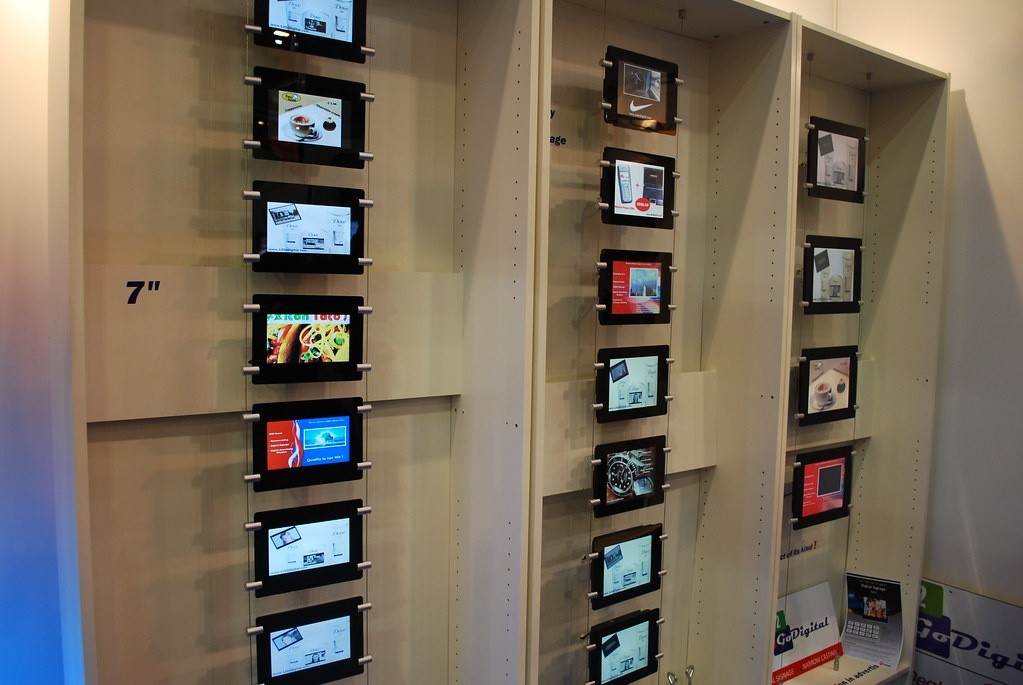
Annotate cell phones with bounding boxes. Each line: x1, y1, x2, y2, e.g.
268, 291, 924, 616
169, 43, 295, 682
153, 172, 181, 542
617, 165, 633, 203
